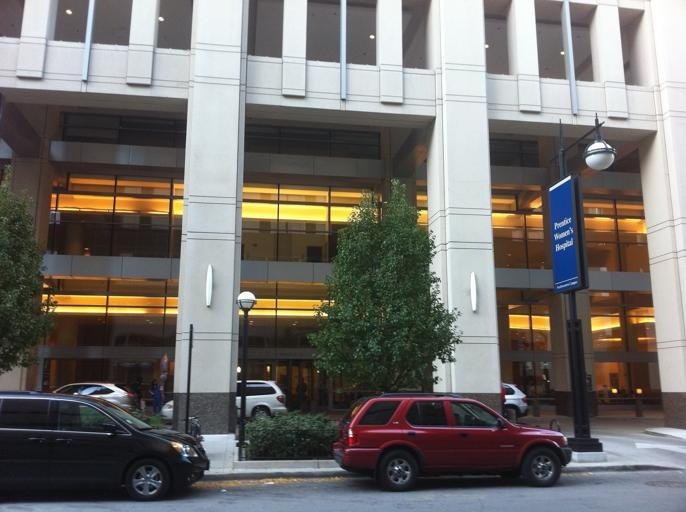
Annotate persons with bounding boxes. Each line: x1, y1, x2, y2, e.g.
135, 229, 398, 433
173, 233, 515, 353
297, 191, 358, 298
146, 376, 162, 411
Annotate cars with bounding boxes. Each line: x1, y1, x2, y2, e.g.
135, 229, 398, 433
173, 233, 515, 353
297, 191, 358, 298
0, 381, 210, 502
331, 383, 573, 492
235, 377, 289, 423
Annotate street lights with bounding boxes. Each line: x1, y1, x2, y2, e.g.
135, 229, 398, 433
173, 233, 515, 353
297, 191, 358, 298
235, 290, 257, 462
548, 112, 618, 453
